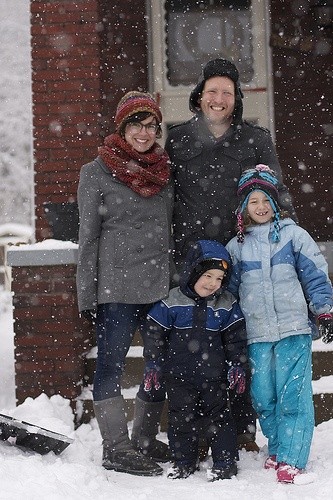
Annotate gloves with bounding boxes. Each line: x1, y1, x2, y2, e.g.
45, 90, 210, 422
227, 366, 246, 394
317, 314, 333, 344
143, 362, 161, 391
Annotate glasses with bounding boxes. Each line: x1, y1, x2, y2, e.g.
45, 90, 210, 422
127, 121, 159, 132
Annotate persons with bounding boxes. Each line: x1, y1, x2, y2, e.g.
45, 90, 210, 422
144, 240, 252, 481
77, 91, 181, 476
224, 164, 333, 483
163, 59, 298, 463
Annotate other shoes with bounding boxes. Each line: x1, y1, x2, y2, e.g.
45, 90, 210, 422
198, 447, 208, 462
237, 441, 260, 454
167, 462, 200, 479
208, 463, 238, 482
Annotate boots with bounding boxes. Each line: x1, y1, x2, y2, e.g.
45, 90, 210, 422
93, 395, 164, 476
131, 395, 171, 463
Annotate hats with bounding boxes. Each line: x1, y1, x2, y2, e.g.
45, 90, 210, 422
234, 164, 281, 244
115, 91, 163, 139
189, 58, 244, 128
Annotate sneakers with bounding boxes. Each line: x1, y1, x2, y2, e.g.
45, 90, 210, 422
276, 463, 302, 482
265, 455, 279, 470
